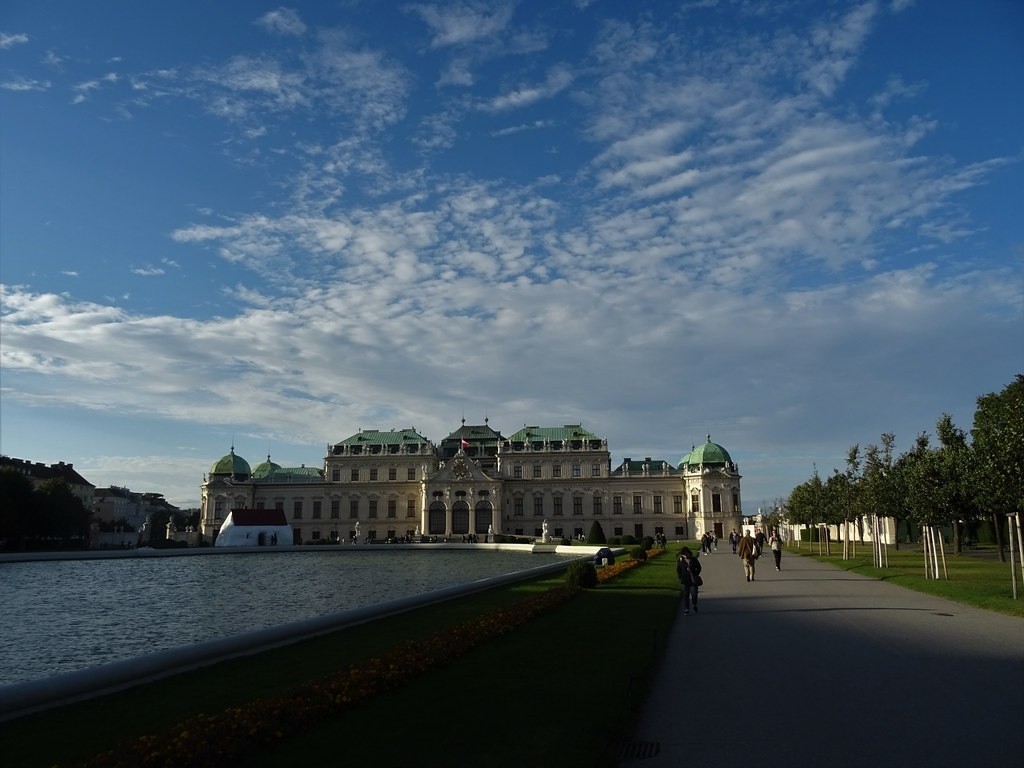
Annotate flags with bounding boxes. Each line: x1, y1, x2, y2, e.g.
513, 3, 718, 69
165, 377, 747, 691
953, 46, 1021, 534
461, 439, 468, 447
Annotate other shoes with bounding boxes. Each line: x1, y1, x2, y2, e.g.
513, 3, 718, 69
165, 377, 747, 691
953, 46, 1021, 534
732, 552, 737, 554
702, 551, 708, 556
759, 555, 762, 558
746, 576, 750, 583
683, 610, 691, 616
709, 552, 713, 556
692, 607, 699, 614
752, 578, 755, 581
775, 566, 780, 571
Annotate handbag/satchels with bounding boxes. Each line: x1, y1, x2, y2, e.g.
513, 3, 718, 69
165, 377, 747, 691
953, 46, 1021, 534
751, 537, 759, 557
689, 573, 703, 587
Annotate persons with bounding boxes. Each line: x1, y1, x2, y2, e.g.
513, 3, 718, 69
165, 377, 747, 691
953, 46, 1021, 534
270, 533, 277, 546
769, 530, 784, 571
462, 533, 479, 543
756, 529, 768, 558
655, 532, 666, 549
578, 534, 585, 542
337, 535, 345, 544
677, 547, 703, 616
562, 533, 564, 540
121, 540, 132, 550
701, 531, 718, 555
484, 534, 488, 543
729, 529, 743, 554
353, 536, 357, 545
738, 530, 760, 582
100, 541, 107, 550
366, 534, 447, 543
